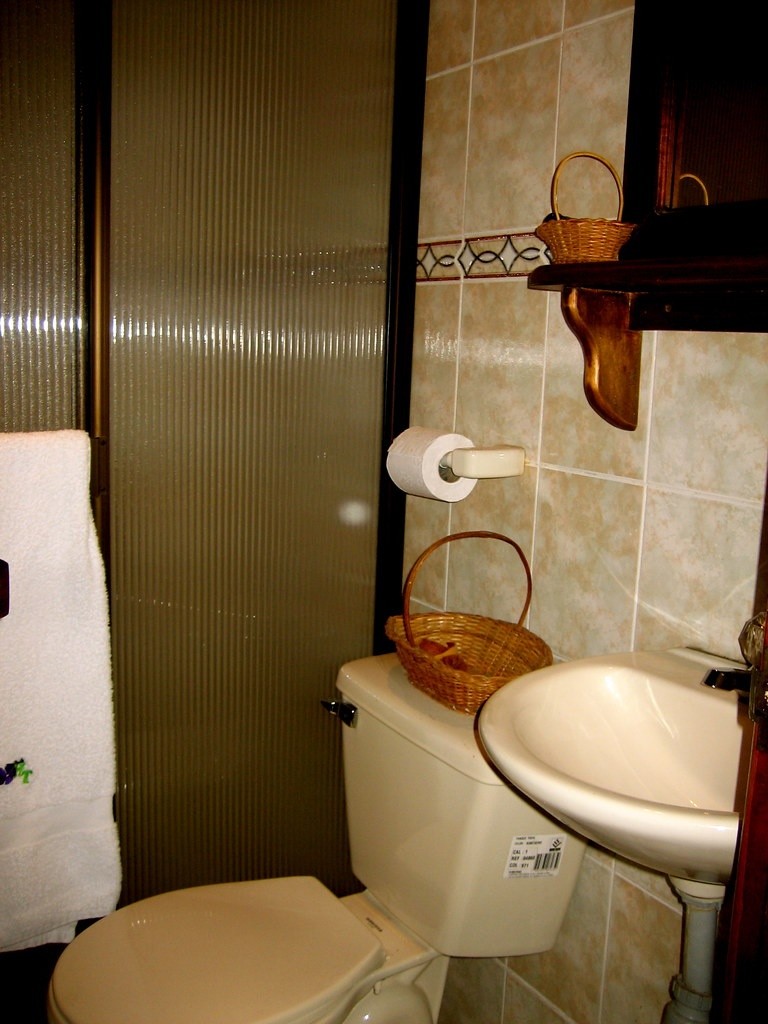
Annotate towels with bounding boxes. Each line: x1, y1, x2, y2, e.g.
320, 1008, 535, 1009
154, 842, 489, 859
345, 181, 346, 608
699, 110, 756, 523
0, 428, 124, 955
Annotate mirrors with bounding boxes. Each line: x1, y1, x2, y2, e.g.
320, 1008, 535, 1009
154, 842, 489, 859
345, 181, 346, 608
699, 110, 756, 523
619, 0, 768, 332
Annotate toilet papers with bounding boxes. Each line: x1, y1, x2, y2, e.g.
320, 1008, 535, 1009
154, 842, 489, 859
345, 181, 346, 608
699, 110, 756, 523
386, 425, 478, 504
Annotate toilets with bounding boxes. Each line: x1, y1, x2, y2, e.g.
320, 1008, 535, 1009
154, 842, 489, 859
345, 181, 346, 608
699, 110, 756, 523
46, 646, 588, 1024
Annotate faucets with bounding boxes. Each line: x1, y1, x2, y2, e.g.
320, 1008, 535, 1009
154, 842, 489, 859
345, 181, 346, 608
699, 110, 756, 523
699, 666, 755, 693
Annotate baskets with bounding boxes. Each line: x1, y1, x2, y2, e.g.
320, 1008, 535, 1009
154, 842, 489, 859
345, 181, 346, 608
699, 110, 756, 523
535, 151, 636, 263
386, 529, 553, 717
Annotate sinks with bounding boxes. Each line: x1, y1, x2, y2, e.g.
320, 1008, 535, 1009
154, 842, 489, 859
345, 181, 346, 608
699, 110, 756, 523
473, 647, 758, 887
